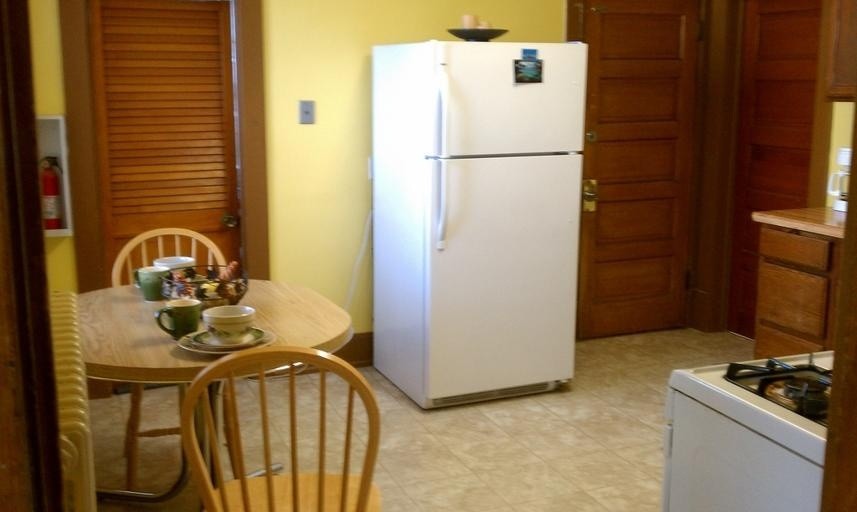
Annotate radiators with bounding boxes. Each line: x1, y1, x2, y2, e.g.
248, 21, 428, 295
47, 288, 98, 512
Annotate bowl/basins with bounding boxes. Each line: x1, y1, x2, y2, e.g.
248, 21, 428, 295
158, 265, 250, 309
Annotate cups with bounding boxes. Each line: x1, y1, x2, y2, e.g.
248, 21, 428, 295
132, 266, 168, 300
153, 256, 196, 268
201, 305, 256, 343
155, 298, 202, 340
460, 15, 477, 30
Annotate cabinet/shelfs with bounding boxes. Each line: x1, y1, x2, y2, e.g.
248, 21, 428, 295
748, 206, 845, 358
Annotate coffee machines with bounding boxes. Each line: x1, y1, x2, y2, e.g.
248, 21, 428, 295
824, 148, 852, 214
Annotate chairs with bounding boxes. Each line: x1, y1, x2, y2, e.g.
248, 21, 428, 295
182, 350, 382, 512
111, 226, 264, 489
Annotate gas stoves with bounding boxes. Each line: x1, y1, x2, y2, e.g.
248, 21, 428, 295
661, 349, 835, 467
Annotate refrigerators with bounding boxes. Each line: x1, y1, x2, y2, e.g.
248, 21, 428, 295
370, 40, 589, 409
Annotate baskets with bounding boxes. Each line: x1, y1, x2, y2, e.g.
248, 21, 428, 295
158, 264, 248, 319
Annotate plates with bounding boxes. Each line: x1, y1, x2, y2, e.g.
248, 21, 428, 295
446, 28, 507, 43
177, 329, 276, 355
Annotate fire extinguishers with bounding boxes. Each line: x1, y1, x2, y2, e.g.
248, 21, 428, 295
39, 157, 63, 229
